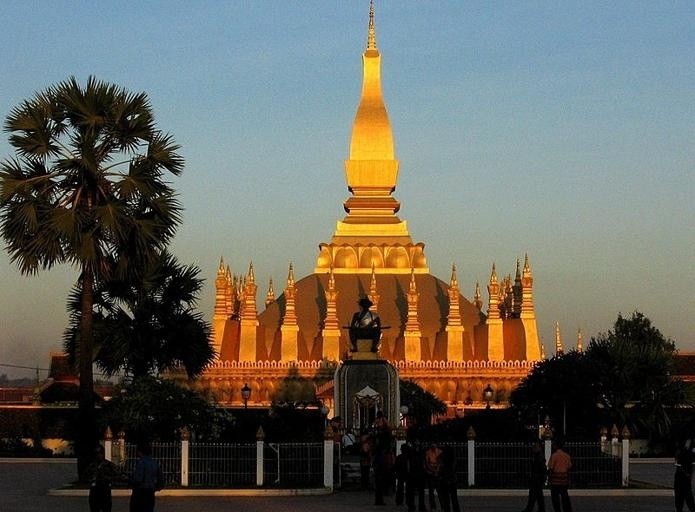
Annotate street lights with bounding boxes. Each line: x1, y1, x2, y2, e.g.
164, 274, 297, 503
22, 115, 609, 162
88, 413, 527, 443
241, 382, 251, 407
483, 382, 494, 409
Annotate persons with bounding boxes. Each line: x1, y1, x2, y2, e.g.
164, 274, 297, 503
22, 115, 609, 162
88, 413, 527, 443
369, 444, 389, 506
341, 429, 357, 456
124, 441, 164, 512
383, 445, 396, 498
405, 442, 428, 511
436, 445, 462, 512
395, 443, 409, 506
425, 439, 444, 512
347, 297, 381, 353
547, 439, 573, 512
672, 439, 695, 512
85, 444, 125, 512
372, 411, 388, 427
359, 435, 375, 492
522, 440, 548, 511
